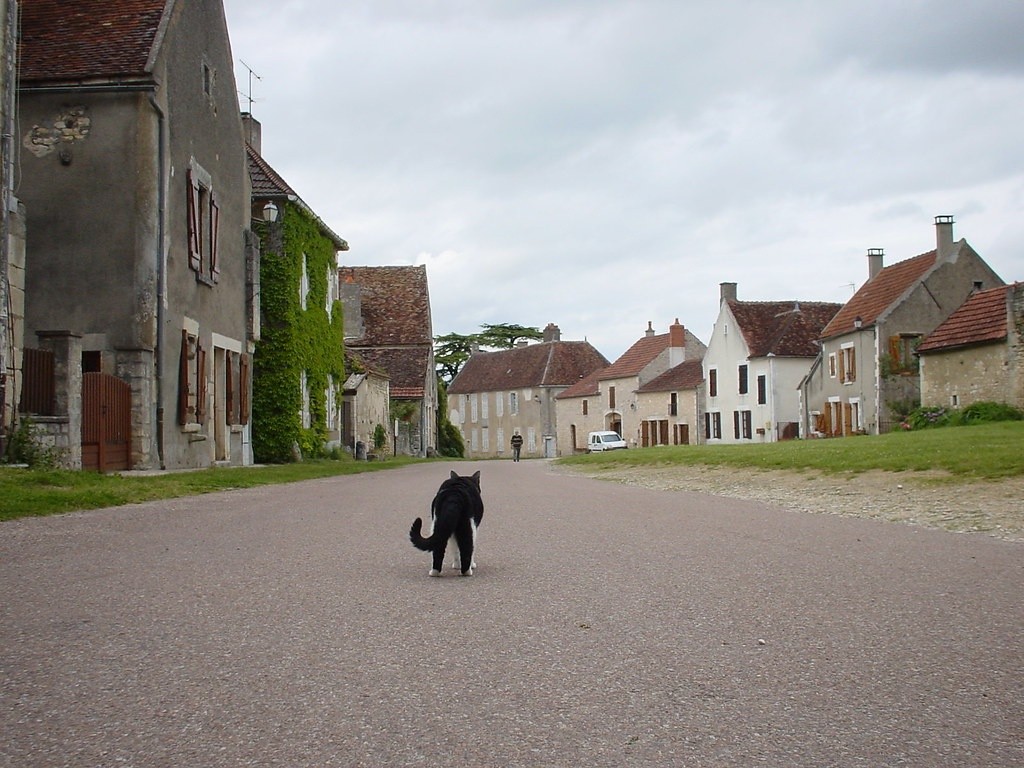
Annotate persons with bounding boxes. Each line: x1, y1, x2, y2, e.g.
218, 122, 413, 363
511, 431, 523, 463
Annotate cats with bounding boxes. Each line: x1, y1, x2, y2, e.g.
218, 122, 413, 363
408, 470, 484, 577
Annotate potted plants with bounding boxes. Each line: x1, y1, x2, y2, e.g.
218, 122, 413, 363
367, 449, 380, 463
372, 424, 388, 461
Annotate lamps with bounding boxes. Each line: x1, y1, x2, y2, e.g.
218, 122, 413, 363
534, 395, 541, 405
251, 200, 278, 226
853, 316, 876, 341
631, 404, 636, 411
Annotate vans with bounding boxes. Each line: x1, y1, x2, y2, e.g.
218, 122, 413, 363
588, 431, 628, 453
